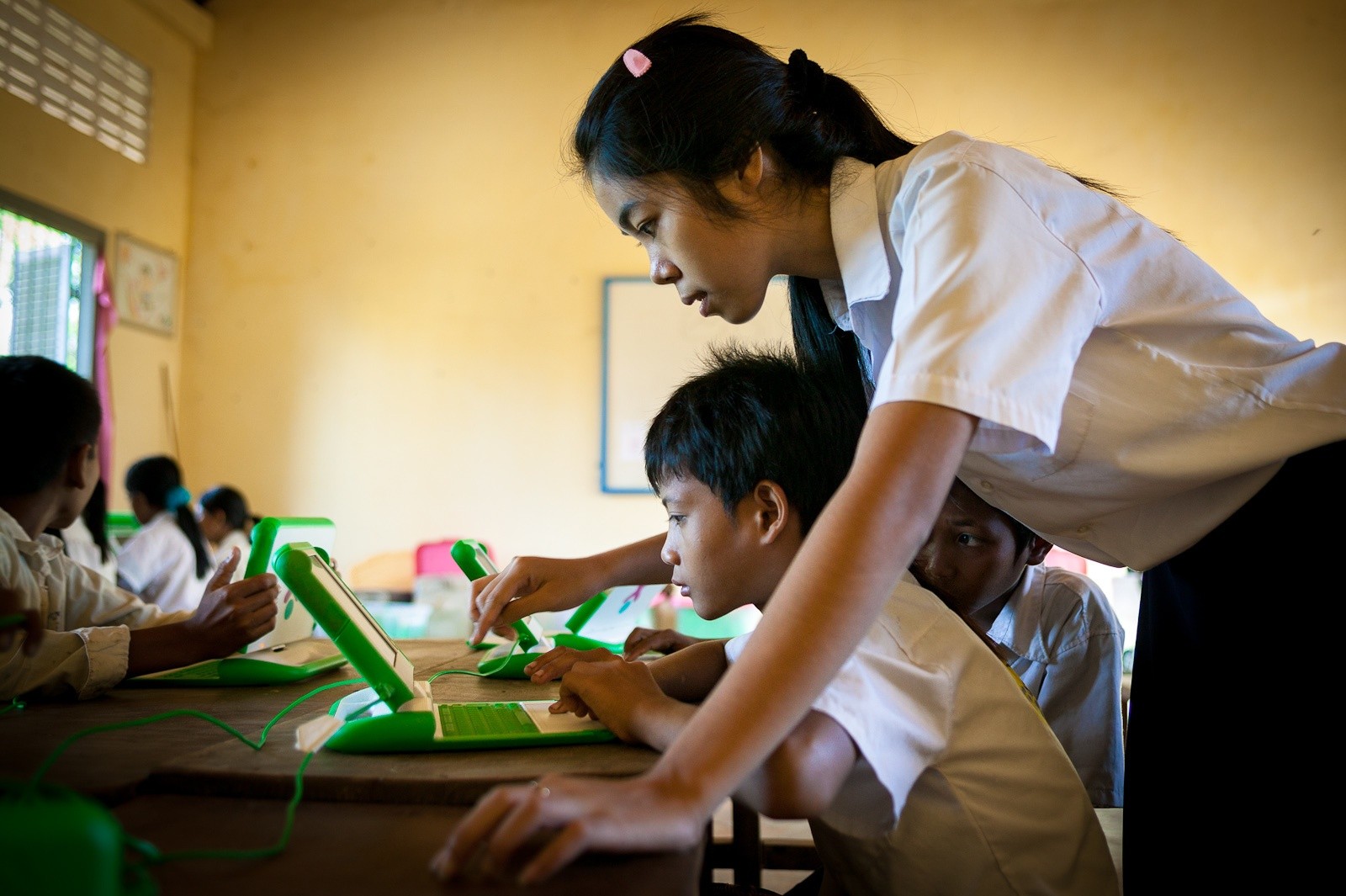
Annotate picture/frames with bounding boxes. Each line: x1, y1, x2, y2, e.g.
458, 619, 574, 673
110, 228, 181, 336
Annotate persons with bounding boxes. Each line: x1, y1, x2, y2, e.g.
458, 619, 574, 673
42, 475, 118, 585
427, 10, 1346, 896
549, 356, 1124, 896
0, 354, 280, 699
197, 484, 264, 585
118, 454, 216, 613
524, 477, 1122, 810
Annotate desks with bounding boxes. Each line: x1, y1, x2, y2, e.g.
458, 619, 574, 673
0, 637, 706, 896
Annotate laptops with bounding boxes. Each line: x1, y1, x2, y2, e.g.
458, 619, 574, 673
103, 512, 668, 755
